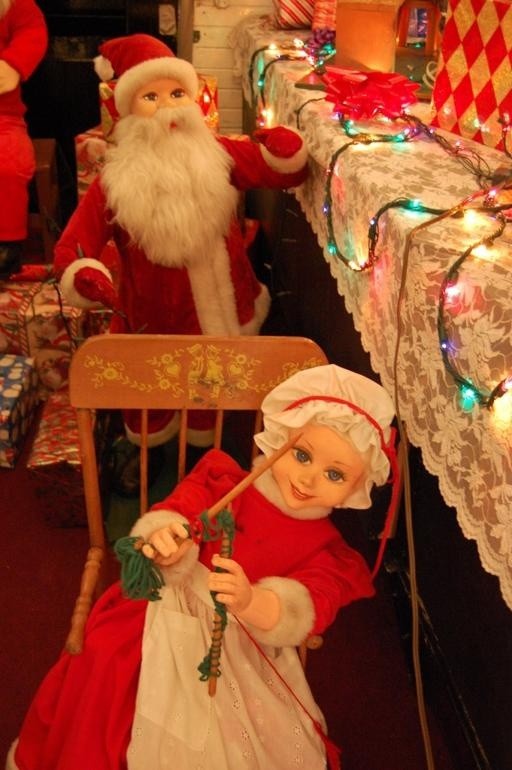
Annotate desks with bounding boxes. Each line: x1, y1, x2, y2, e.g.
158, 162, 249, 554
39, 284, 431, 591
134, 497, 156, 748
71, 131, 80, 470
241, 12, 512, 768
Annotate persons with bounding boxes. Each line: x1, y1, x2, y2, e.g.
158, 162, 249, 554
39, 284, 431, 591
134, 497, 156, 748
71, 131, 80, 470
1, 0, 50, 252
2, 362, 398, 770
48, 31, 311, 503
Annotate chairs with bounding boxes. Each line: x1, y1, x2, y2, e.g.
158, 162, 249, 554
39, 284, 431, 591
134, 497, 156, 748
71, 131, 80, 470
66, 335, 332, 675
